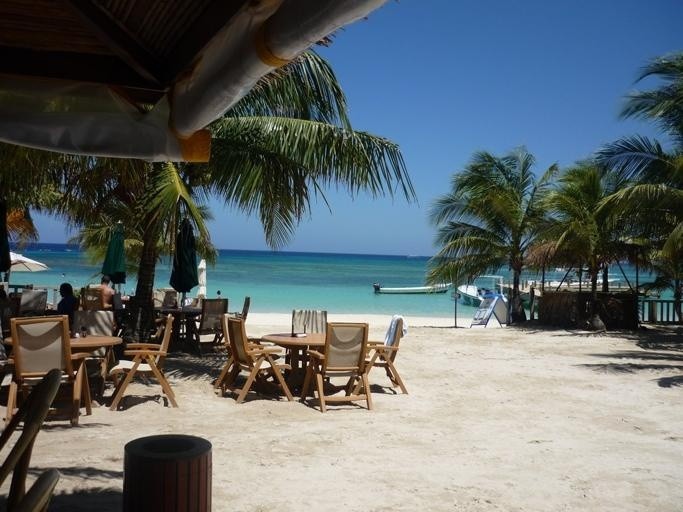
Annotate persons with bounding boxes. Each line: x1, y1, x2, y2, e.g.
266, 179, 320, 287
96, 274, 115, 309
44, 281, 75, 328
215, 289, 223, 299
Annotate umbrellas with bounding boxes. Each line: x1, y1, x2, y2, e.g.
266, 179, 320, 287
196, 256, 208, 303
100, 220, 126, 292
166, 217, 198, 307
7, 250, 50, 285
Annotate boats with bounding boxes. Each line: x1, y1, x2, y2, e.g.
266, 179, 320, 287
457, 284, 495, 305
373, 282, 453, 294
527, 278, 620, 289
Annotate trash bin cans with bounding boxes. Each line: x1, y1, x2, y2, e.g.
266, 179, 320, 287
122, 434, 213, 512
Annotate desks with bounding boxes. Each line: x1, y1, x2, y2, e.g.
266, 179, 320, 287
4, 335, 122, 407
261, 333, 329, 388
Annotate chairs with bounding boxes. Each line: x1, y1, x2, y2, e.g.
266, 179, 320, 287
0, 368, 63, 511
345, 314, 407, 395
214, 312, 295, 404
0, 284, 251, 359
109, 314, 179, 412
286, 308, 330, 386
5, 314, 91, 428
300, 322, 373, 414
